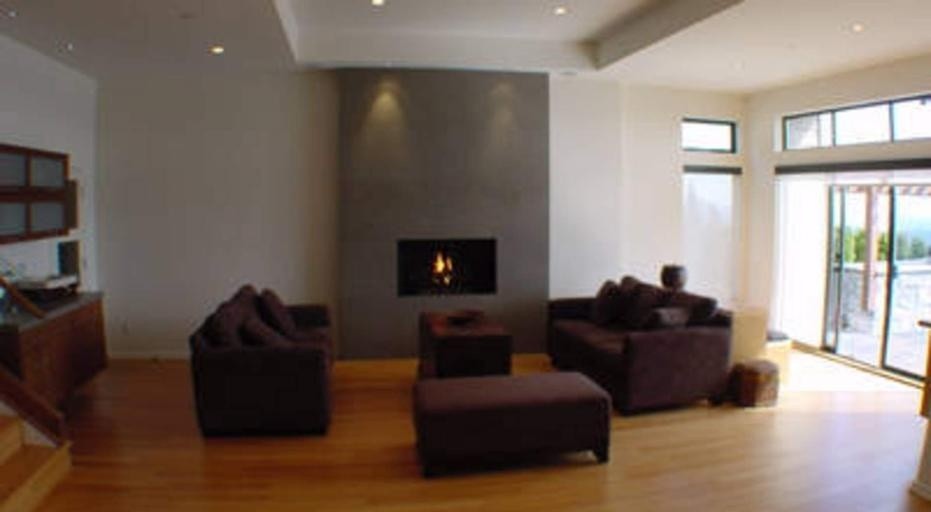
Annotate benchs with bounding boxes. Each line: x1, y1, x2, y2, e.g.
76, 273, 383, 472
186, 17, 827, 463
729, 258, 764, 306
412, 370, 611, 478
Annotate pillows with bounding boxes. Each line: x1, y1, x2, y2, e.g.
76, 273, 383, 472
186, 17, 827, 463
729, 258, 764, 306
593, 280, 628, 325
257, 288, 296, 340
640, 307, 693, 329
239, 316, 289, 344
621, 284, 670, 329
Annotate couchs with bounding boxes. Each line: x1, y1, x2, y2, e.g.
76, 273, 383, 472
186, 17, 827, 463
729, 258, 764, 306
546, 274, 734, 416
189, 283, 330, 438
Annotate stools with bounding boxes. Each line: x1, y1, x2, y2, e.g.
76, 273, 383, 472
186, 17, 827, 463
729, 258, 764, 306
736, 358, 781, 409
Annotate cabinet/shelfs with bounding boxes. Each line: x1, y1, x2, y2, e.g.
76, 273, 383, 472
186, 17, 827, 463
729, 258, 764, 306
0, 291, 107, 407
